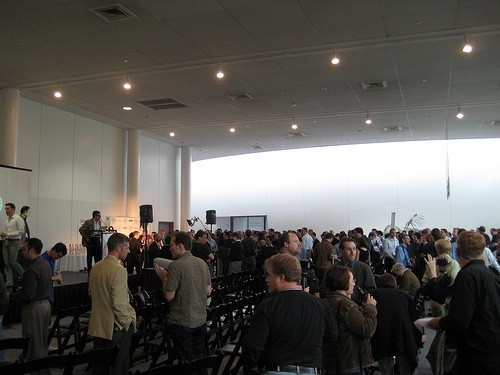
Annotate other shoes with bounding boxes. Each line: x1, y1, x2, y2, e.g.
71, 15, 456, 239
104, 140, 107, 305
47, 346, 58, 352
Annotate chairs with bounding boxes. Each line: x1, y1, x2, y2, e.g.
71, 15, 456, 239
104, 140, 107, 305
0, 262, 446, 375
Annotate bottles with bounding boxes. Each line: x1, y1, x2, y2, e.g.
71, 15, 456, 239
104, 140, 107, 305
57, 269, 62, 284
356, 285, 368, 306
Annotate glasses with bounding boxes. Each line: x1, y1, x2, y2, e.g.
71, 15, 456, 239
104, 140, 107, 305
351, 279, 356, 283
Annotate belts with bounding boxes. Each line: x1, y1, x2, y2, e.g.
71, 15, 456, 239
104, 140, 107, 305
265, 364, 323, 375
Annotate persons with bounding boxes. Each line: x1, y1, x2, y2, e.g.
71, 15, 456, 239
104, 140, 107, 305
156, 231, 211, 375
320, 237, 377, 307
0, 272, 8, 361
88, 233, 137, 375
0, 203, 24, 290
320, 265, 377, 375
127, 230, 180, 275
428, 231, 500, 375
206, 228, 283, 276
280, 231, 301, 255
295, 226, 370, 296
79, 211, 103, 276
245, 254, 337, 375
370, 273, 418, 375
477, 226, 500, 275
19, 206, 30, 264
15, 238, 51, 375
369, 228, 465, 347
393, 264, 419, 300
40, 242, 67, 349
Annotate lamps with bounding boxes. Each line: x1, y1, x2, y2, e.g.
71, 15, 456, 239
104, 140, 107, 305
462, 35, 473, 53
366, 113, 372, 125
455, 105, 463, 118
186, 216, 206, 230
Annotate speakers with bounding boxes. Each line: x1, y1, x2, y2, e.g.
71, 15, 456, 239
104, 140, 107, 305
139, 204, 153, 223
206, 210, 216, 224
